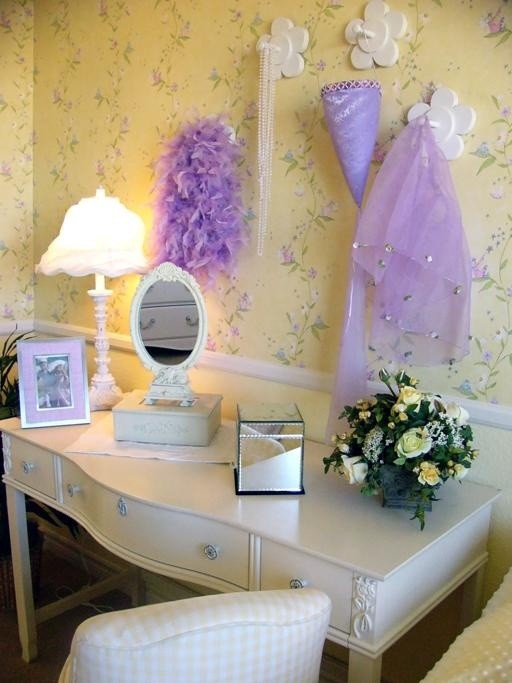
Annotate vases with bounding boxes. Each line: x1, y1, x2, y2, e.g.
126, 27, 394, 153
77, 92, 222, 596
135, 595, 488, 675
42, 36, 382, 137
379, 465, 434, 513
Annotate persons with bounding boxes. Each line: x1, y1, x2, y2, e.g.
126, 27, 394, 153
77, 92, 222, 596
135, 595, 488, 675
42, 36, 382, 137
35, 357, 70, 408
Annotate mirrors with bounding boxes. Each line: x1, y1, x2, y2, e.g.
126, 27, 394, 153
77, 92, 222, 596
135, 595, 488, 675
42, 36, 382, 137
129, 263, 208, 377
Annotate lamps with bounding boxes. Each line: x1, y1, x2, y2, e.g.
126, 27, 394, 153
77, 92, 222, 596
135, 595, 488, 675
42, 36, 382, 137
34, 185, 156, 412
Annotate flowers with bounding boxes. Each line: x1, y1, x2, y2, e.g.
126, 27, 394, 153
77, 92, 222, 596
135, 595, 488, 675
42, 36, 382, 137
322, 368, 478, 531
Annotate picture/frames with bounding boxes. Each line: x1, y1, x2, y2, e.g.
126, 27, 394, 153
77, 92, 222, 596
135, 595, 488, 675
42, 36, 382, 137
17, 336, 92, 429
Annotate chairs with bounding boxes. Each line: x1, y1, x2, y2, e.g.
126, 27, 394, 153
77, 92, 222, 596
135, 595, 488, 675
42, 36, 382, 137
57, 587, 333, 683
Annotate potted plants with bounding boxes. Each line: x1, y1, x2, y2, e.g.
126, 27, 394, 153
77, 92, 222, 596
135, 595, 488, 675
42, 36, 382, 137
1, 322, 85, 616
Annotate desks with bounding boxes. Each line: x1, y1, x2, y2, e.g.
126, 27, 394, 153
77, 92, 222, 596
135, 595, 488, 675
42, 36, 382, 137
0, 405, 506, 682
